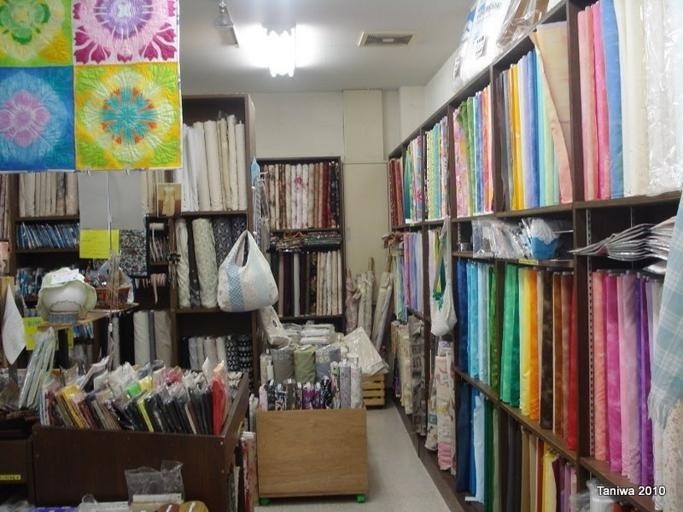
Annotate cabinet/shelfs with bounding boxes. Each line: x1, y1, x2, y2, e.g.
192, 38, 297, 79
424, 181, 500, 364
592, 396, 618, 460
23, 371, 254, 511
1, 92, 261, 395
386, 0, 683, 512
255, 157, 347, 335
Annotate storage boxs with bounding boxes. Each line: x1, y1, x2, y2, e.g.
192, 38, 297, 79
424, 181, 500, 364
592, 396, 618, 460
255, 406, 373, 503
